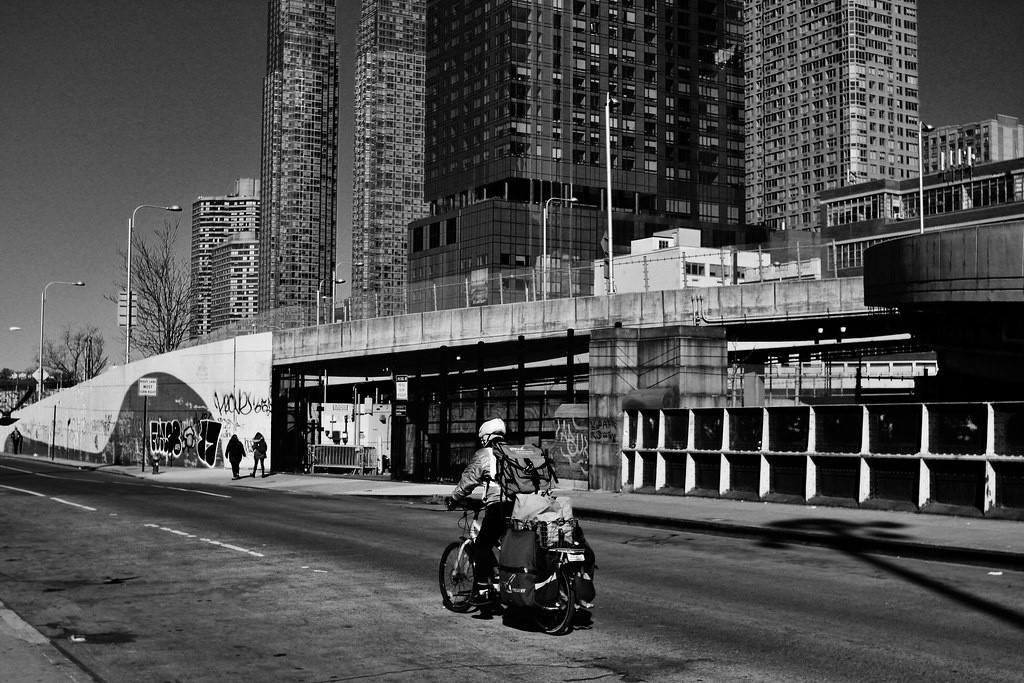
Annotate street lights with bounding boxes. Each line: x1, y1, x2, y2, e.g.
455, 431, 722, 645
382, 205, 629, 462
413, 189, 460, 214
37, 281, 85, 402
543, 198, 578, 300
603, 92, 620, 293
917, 122, 935, 233
332, 262, 365, 324
126, 205, 184, 365
317, 278, 346, 326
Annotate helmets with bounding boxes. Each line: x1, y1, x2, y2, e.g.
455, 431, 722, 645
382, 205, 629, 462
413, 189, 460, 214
477, 417, 507, 448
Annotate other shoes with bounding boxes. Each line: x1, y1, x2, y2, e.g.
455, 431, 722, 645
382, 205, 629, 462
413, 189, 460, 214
250, 473, 255, 478
231, 475, 241, 480
262, 474, 265, 478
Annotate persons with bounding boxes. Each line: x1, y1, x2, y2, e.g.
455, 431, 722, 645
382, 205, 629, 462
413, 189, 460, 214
252, 432, 267, 478
11, 428, 21, 454
225, 435, 246, 480
444, 418, 518, 608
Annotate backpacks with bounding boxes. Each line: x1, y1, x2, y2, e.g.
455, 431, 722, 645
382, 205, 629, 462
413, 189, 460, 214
491, 441, 560, 498
256, 440, 268, 454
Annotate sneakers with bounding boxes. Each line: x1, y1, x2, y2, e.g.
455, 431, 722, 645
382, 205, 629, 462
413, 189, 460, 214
467, 580, 495, 606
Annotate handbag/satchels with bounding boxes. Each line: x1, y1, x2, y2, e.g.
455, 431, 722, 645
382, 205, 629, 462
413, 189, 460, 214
511, 489, 576, 548
570, 520, 600, 610
498, 527, 569, 611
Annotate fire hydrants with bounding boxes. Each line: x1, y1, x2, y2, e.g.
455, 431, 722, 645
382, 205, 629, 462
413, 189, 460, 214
151, 456, 160, 473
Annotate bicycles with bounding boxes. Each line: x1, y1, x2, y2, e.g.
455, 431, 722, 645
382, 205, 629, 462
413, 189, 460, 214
438, 497, 586, 634
